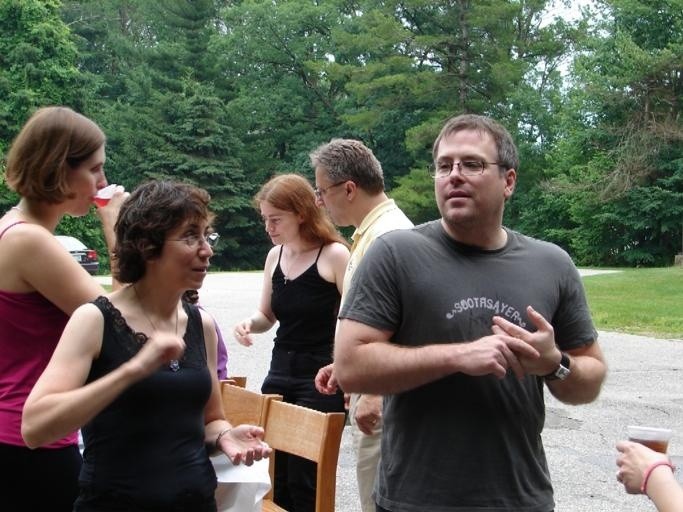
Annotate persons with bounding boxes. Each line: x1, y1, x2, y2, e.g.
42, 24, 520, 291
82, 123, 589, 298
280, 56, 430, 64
21, 179, 272, 512
332, 112, 608, 512
232, 174, 350, 512
614, 440, 683, 512
307, 137, 416, 512
182, 289, 228, 380
0, 105, 130, 512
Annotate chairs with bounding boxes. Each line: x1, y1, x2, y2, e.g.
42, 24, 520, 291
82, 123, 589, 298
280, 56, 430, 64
219, 373, 346, 512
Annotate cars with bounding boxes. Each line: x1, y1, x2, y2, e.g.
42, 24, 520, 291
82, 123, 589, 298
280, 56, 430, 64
50, 234, 99, 275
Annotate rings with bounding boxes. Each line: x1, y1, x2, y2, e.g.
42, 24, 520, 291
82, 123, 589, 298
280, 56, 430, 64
235, 333, 239, 336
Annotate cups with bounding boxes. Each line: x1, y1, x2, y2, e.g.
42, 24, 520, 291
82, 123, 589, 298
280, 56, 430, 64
620, 424, 675, 494
88, 182, 117, 208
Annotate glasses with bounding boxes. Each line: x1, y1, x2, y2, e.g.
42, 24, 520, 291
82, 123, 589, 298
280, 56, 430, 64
165, 233, 220, 250
313, 181, 345, 201
426, 161, 497, 178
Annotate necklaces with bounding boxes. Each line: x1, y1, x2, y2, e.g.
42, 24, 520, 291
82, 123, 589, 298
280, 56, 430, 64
132, 282, 180, 373
282, 236, 303, 284
12, 206, 24, 211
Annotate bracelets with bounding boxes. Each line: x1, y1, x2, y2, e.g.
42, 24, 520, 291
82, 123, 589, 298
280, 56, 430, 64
640, 461, 677, 495
215, 428, 232, 452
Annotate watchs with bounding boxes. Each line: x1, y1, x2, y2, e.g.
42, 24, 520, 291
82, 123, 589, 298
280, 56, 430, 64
542, 349, 571, 382
